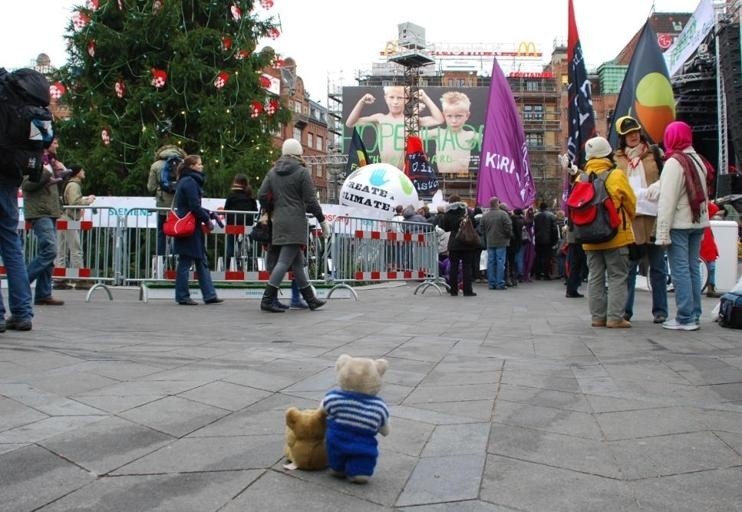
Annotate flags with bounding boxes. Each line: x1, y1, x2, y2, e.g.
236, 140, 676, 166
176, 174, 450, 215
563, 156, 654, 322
567, 0, 598, 203
608, 17, 676, 157
476, 58, 536, 282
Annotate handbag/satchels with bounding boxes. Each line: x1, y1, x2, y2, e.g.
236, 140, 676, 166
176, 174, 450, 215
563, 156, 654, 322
521, 226, 531, 243
454, 217, 481, 245
163, 209, 196, 237
718, 290, 742, 329
251, 223, 272, 242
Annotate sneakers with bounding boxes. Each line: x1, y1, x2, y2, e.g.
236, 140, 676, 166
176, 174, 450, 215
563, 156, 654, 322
180, 298, 198, 305
0, 316, 31, 332
35, 295, 64, 305
68, 163, 82, 177
75, 279, 94, 289
449, 289, 458, 296
205, 297, 223, 304
464, 291, 476, 296
654, 311, 701, 331
592, 312, 633, 328
53, 279, 73, 290
475, 274, 549, 289
566, 288, 583, 297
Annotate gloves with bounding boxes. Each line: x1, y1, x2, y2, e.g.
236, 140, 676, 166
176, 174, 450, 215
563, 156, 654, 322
645, 181, 660, 200
319, 220, 333, 238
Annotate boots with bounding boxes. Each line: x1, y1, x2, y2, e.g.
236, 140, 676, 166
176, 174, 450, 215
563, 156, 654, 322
261, 280, 327, 312
707, 283, 721, 297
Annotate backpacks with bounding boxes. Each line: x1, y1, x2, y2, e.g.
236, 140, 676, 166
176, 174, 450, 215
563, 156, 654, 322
566, 169, 622, 244
0, 68, 55, 188
157, 155, 182, 193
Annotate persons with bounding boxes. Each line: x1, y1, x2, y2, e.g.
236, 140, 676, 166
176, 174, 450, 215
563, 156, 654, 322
262, 192, 309, 310
147, 145, 187, 263
654, 121, 711, 331
256, 138, 332, 312
0, 67, 95, 329
170, 154, 225, 305
564, 136, 635, 328
391, 192, 587, 297
403, 91, 480, 174
699, 202, 723, 298
345, 86, 445, 172
611, 115, 669, 325
217, 173, 257, 273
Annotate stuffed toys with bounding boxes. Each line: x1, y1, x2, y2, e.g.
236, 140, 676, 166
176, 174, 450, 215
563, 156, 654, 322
285, 404, 328, 471
321, 354, 392, 482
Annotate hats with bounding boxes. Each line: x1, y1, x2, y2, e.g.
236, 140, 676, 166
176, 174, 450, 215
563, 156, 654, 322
585, 136, 613, 161
615, 117, 641, 135
281, 138, 303, 156
